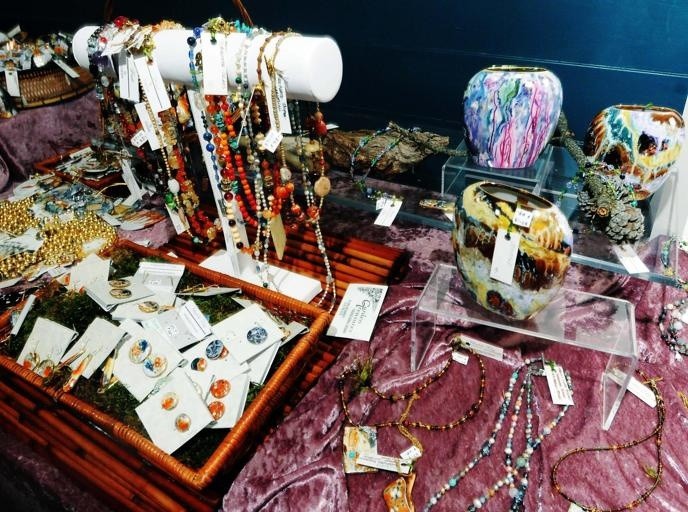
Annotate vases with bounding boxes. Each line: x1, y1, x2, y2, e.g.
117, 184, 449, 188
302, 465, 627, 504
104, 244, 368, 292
460, 66, 564, 170
451, 180, 573, 323
583, 105, 685, 200
0, 162, 688, 512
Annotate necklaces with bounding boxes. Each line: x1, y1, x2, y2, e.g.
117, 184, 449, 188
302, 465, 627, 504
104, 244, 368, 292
335, 338, 667, 512
85, 18, 337, 315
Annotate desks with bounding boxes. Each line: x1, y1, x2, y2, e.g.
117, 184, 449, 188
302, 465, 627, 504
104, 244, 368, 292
0, 88, 104, 193
